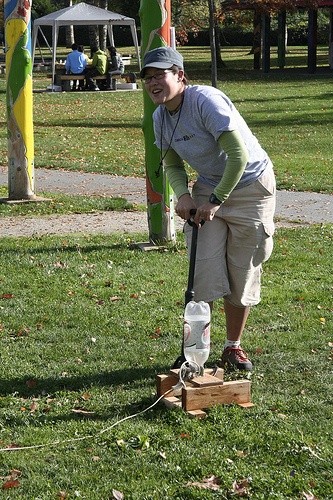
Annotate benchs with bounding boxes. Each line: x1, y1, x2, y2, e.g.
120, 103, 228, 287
60, 75, 121, 91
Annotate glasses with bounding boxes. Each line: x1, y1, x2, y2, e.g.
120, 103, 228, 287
142, 72, 167, 84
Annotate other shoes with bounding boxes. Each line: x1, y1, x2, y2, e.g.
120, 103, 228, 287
70, 86, 83, 91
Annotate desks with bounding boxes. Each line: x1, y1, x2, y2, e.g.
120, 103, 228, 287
55, 61, 94, 86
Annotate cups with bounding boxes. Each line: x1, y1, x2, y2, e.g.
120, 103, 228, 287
60, 59, 63, 63
64, 59, 66, 63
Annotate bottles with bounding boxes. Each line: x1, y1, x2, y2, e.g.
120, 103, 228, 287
183, 300, 211, 375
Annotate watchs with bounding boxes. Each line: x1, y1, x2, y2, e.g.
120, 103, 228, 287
209, 193, 222, 206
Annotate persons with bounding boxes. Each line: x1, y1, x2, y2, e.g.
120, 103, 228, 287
65, 41, 125, 91
139, 47, 277, 371
125, 73, 138, 89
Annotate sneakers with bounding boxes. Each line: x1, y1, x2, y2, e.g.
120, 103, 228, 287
221, 346, 253, 371
94, 87, 100, 90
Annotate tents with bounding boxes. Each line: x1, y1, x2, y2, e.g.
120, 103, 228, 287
31, 2, 141, 93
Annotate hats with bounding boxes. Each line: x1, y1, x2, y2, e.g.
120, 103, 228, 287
140, 47, 184, 77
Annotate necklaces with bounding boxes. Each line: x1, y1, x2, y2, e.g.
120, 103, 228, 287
153, 93, 184, 176
